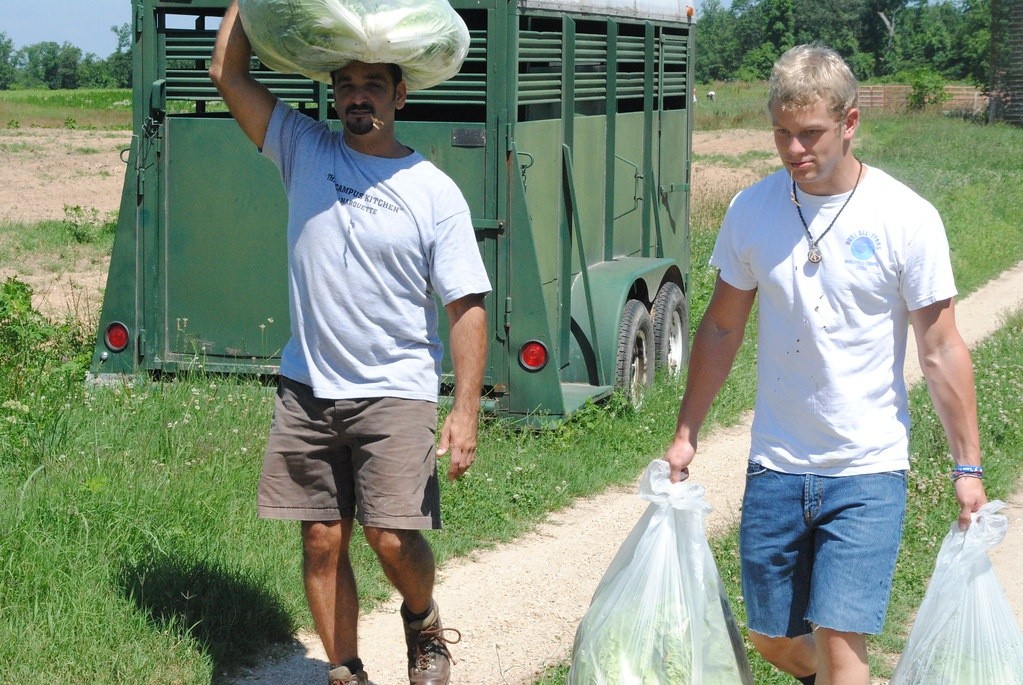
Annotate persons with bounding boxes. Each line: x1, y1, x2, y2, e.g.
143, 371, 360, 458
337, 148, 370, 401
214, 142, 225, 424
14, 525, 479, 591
654, 44, 989, 685
204, 0, 495, 685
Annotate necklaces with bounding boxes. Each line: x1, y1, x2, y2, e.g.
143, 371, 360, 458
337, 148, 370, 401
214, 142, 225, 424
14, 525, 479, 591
792, 156, 862, 263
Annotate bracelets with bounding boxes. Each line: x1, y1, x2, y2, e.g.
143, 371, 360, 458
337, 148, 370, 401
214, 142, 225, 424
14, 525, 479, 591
951, 465, 984, 482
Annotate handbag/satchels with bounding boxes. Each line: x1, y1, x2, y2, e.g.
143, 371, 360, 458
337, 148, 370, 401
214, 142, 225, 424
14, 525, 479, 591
891, 498, 1022, 685
568, 455, 752, 685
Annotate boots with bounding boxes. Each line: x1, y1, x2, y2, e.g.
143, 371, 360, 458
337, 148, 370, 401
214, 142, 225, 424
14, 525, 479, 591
403, 615, 449, 685
328, 664, 369, 685
